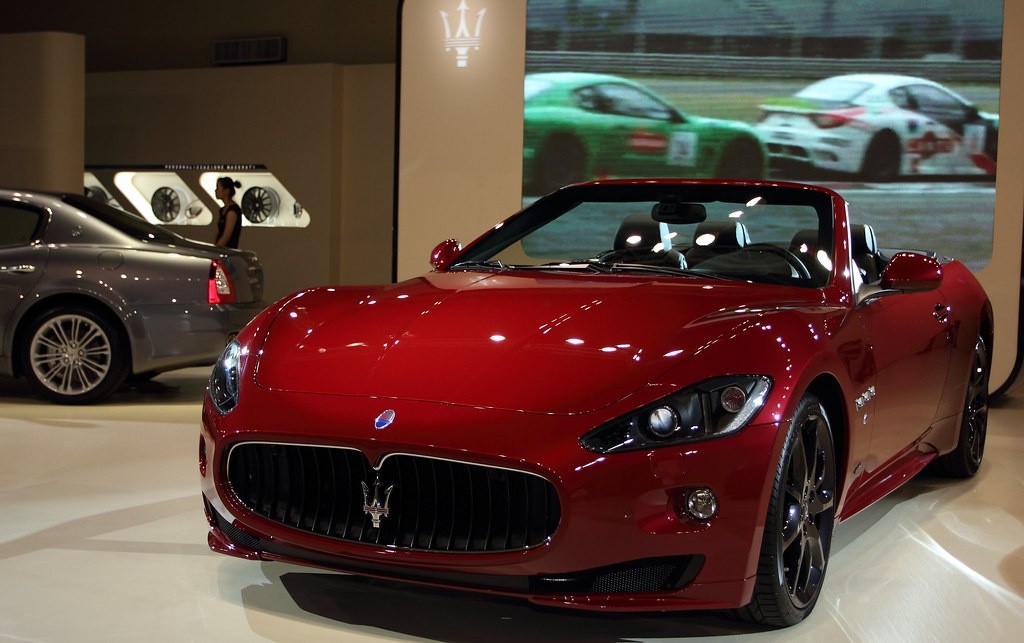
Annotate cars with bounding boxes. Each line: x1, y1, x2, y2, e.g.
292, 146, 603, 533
523, 73, 769, 195
0, 187, 265, 405
754, 74, 1000, 177
198, 178, 995, 627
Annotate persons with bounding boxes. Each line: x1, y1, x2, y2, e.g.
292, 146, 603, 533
213, 178, 243, 250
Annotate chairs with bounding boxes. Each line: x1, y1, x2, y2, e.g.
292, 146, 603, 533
603, 213, 687, 270
789, 230, 819, 275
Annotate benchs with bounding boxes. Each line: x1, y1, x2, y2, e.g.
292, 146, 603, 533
685, 219, 878, 282
